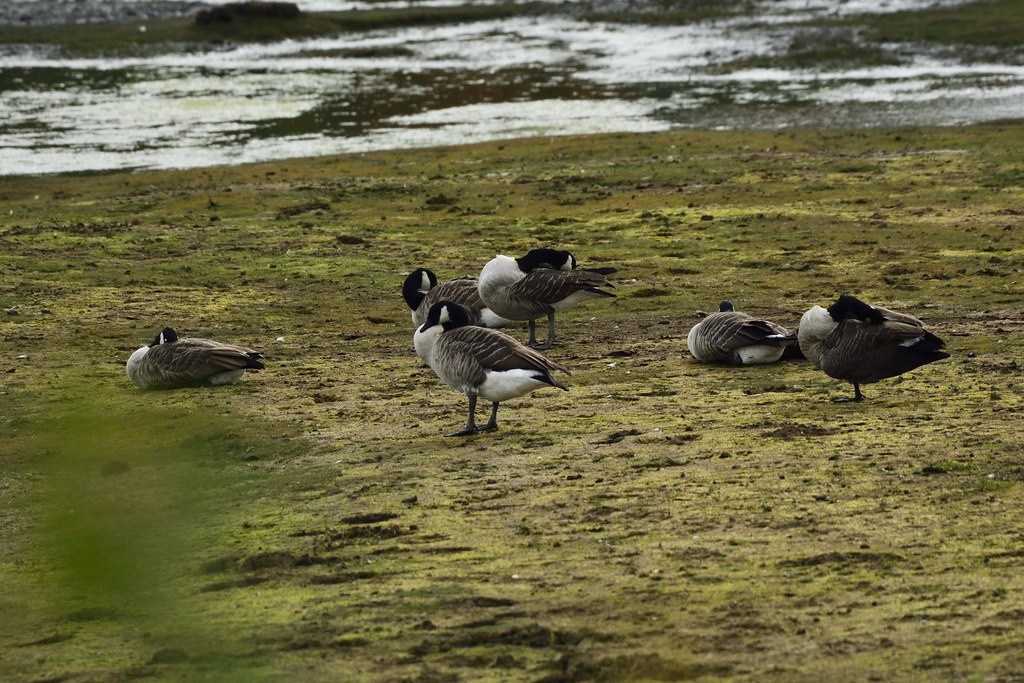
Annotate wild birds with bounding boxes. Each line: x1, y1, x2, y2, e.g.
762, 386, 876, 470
125, 326, 267, 389
797, 295, 951, 404
687, 299, 798, 364
419, 301, 572, 438
402, 268, 512, 330
478, 249, 619, 349
